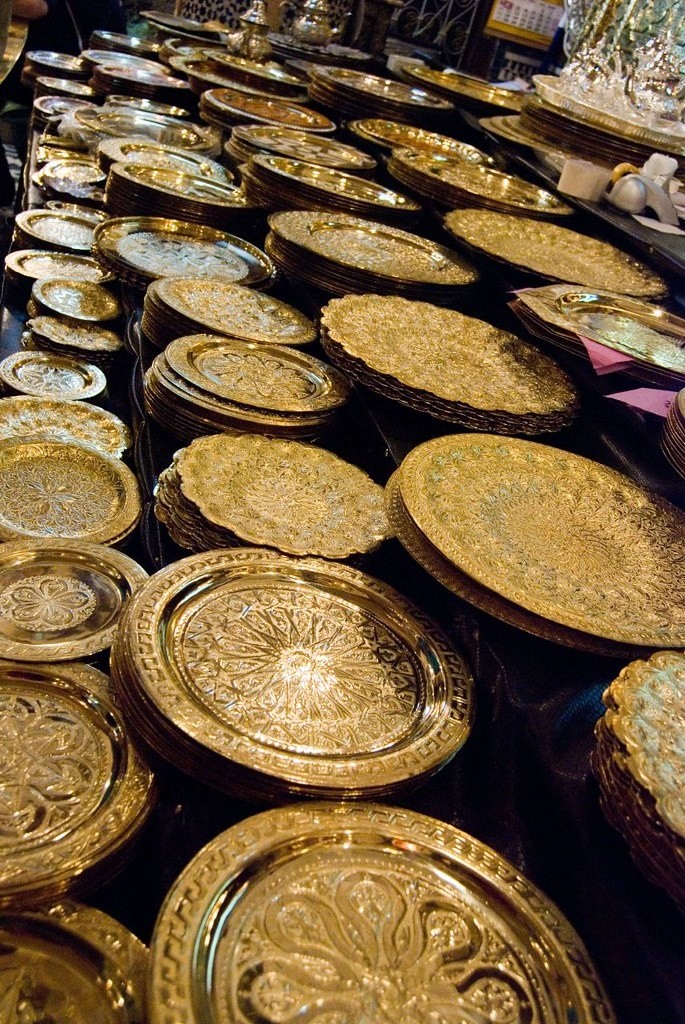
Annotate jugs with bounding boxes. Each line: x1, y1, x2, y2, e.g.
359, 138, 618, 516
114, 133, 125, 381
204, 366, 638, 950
227, 0, 273, 64
276, 0, 352, 46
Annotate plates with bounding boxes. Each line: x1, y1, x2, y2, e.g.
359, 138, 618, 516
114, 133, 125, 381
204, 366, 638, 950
0, 11, 685, 1024
